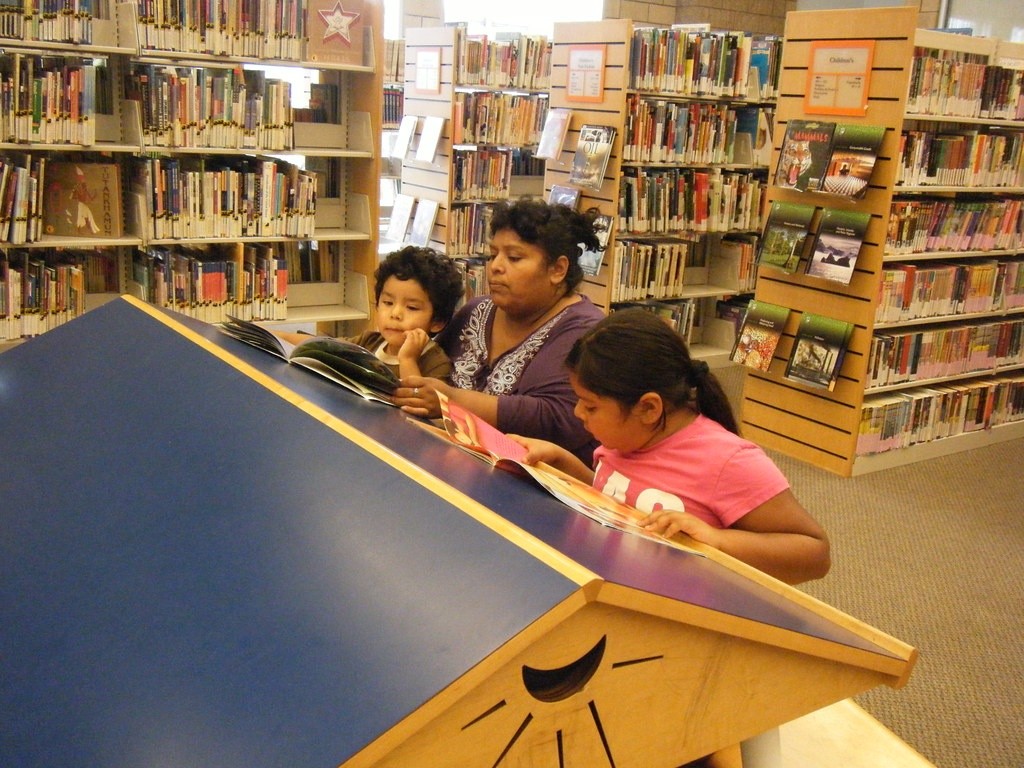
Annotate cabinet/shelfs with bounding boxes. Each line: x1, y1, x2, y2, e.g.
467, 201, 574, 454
380, 7, 1024, 478
0, 0, 384, 350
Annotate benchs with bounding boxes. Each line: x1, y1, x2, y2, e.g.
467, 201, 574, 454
740, 697, 937, 768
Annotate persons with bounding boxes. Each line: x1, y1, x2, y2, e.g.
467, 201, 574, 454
502, 307, 831, 586
258, 247, 462, 389
390, 206, 610, 471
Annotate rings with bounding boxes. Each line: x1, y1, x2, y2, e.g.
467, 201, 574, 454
413, 387, 419, 396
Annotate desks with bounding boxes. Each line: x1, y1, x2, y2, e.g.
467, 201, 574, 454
0, 295, 920, 768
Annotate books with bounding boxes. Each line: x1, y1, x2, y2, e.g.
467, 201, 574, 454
0, 0, 344, 341
406, 388, 706, 559
218, 314, 396, 407
381, 22, 1024, 457
